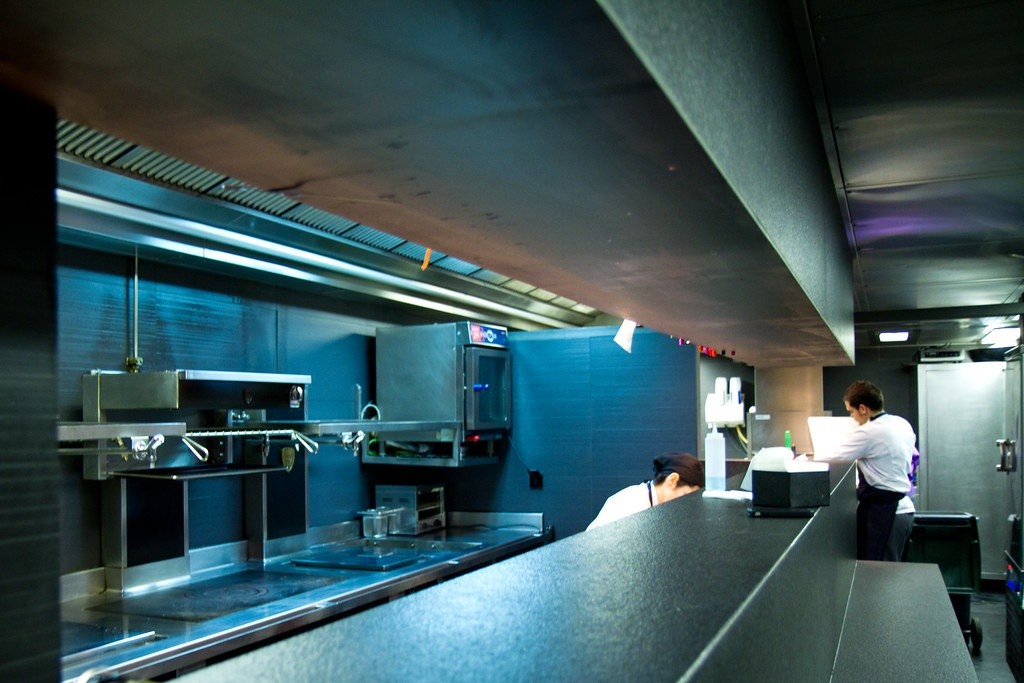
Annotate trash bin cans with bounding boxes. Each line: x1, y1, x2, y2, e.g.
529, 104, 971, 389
905, 511, 983, 648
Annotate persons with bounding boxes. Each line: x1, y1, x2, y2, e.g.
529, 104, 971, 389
584, 453, 705, 531
817, 379, 916, 561
885, 496, 916, 561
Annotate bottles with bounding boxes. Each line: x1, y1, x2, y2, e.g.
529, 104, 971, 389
784, 429, 796, 457
714, 376, 741, 408
704, 422, 726, 492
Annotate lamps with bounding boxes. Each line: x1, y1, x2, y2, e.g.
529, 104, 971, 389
878, 328, 911, 345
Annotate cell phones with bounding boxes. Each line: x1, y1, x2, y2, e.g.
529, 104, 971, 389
746, 506, 814, 520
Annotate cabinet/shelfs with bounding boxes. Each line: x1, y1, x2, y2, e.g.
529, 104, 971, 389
0, 0, 979, 683
